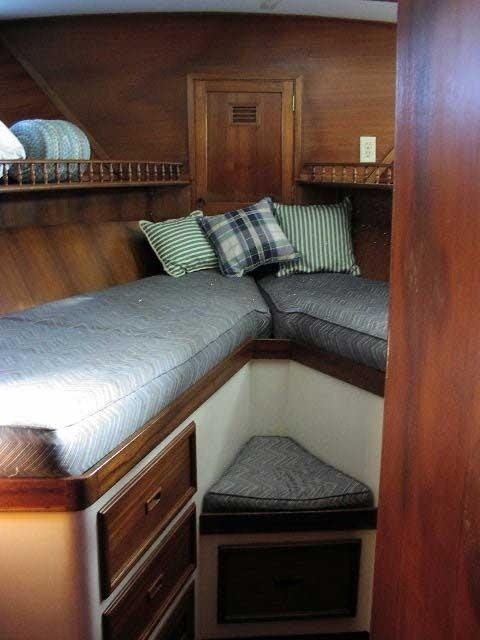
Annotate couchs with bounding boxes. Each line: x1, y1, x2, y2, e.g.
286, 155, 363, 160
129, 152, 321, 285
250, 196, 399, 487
0, 209, 389, 640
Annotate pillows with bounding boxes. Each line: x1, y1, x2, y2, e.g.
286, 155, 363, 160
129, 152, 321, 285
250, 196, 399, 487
272, 196, 362, 280
199, 197, 300, 276
140, 210, 215, 272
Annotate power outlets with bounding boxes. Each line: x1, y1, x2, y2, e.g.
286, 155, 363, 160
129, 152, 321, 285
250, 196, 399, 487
361, 138, 376, 163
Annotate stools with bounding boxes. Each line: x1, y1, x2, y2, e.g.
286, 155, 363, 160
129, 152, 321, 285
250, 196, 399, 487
195, 436, 379, 632
